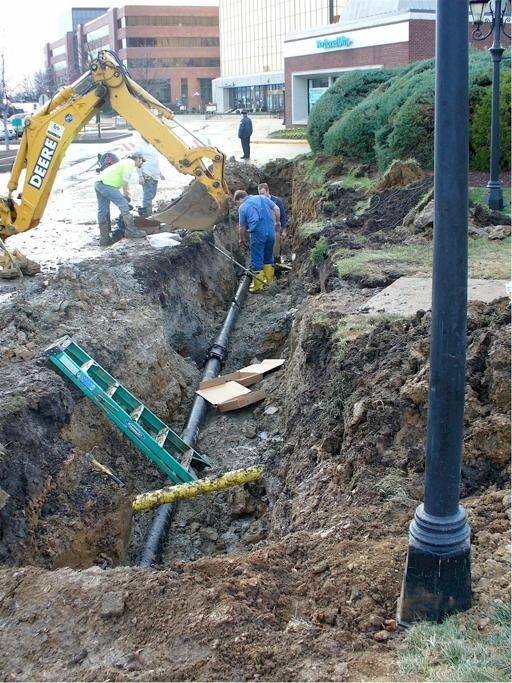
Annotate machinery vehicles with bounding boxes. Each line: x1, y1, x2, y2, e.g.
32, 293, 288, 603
0, 50, 230, 279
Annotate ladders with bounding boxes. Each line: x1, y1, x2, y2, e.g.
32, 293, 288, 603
44, 334, 212, 485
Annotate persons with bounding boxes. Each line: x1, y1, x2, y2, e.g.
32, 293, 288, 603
238, 111, 253, 159
234, 190, 281, 294
135, 138, 165, 216
258, 183, 287, 276
95, 152, 148, 246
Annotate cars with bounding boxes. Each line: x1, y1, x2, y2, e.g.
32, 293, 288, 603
0, 113, 27, 141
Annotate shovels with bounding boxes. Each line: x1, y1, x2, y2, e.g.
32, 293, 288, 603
208, 241, 281, 297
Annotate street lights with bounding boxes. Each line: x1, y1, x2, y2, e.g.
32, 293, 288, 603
469, 0, 511, 208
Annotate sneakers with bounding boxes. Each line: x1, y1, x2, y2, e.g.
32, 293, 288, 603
240, 155, 250, 159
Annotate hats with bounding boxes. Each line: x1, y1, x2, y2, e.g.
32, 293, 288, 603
130, 150, 146, 162
240, 111, 247, 115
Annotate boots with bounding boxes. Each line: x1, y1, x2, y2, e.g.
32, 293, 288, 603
99, 224, 114, 245
123, 214, 146, 238
249, 265, 274, 292
